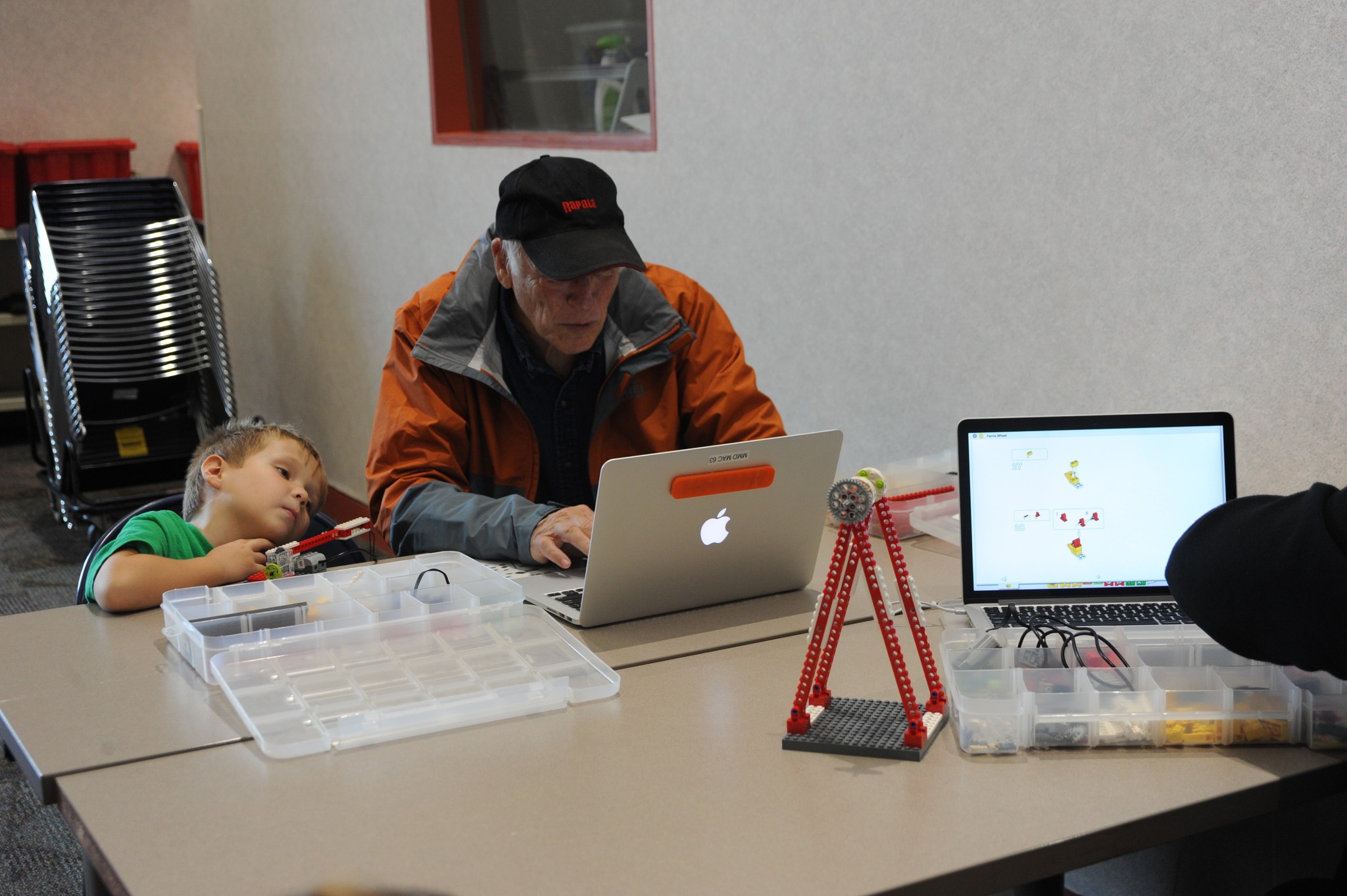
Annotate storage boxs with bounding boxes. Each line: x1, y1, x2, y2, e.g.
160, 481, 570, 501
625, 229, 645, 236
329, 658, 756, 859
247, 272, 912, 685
18, 136, 136, 196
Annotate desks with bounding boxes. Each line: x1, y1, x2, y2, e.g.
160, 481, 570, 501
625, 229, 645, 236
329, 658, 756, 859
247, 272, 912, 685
0, 463, 1347, 896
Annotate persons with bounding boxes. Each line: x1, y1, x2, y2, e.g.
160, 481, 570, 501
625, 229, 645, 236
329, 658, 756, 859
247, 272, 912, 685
1165, 481, 1347, 681
366, 155, 786, 567
85, 417, 327, 611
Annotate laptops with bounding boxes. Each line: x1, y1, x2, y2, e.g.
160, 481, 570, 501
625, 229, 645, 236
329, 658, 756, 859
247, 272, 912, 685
511, 429, 844, 627
958, 410, 1237, 648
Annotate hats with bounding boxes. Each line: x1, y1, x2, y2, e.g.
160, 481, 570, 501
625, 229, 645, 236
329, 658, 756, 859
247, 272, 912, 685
496, 155, 648, 280
